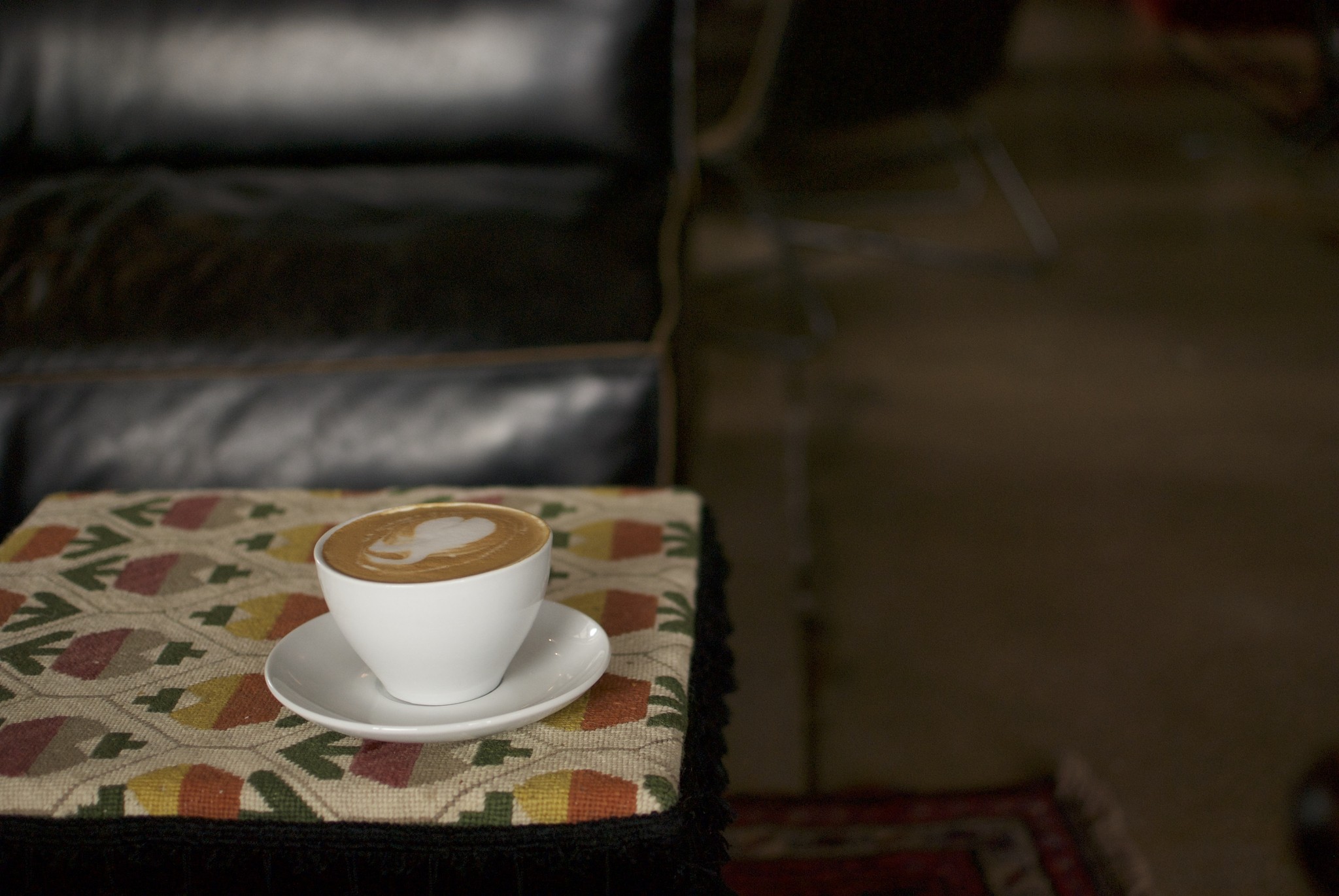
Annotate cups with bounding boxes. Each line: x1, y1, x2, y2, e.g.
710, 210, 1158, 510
313, 503, 555, 705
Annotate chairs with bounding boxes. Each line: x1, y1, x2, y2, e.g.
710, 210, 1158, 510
0, 0, 706, 546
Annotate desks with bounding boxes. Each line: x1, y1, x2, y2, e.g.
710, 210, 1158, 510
0, 486, 732, 896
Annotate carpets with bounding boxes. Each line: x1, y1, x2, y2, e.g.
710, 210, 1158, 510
714, 750, 1153, 895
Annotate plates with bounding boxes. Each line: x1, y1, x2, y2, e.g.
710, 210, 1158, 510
262, 599, 611, 742
